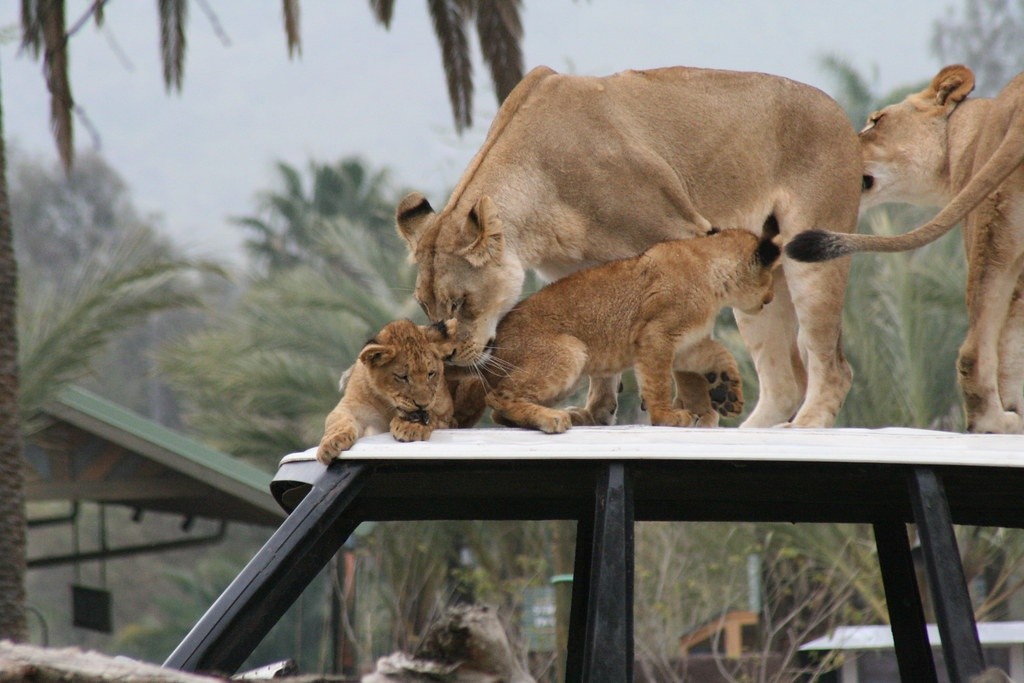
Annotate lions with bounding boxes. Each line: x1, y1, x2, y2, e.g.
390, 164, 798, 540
312, 62, 1024, 467
